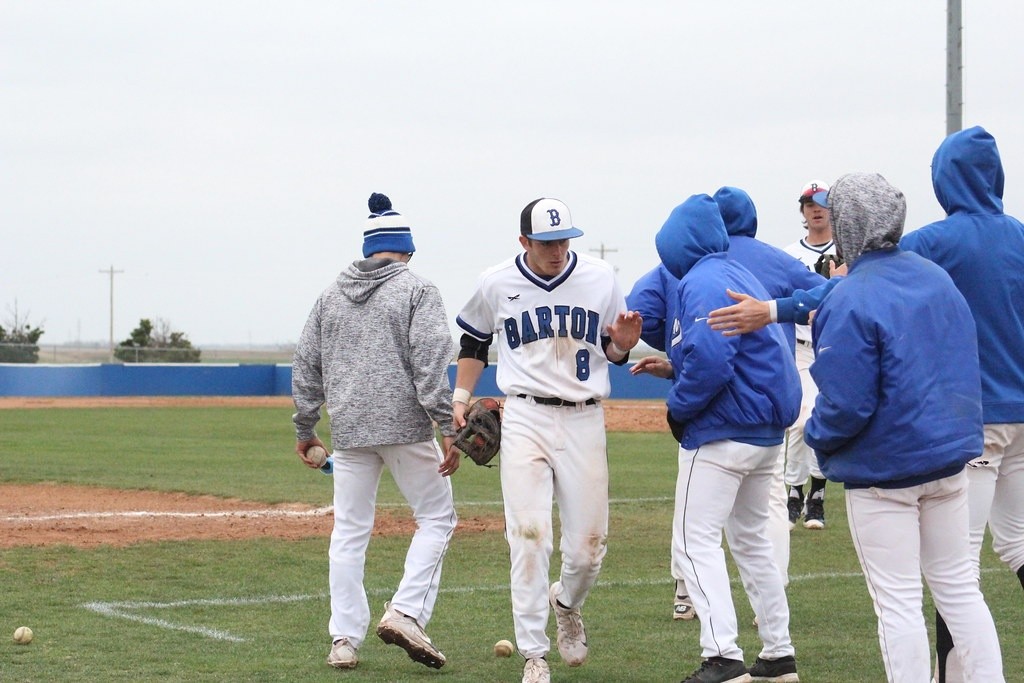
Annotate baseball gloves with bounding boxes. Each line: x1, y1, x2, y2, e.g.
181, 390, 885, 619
452, 398, 503, 467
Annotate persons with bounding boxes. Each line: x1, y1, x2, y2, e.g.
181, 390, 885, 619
451, 198, 642, 683
291, 192, 460, 670
626, 181, 847, 683
706, 124, 1024, 683
803, 172, 1006, 683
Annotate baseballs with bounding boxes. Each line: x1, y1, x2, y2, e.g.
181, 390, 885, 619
304, 444, 328, 468
13, 626, 34, 644
495, 640, 513, 657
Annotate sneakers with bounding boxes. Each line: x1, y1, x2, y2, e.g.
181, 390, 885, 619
549, 582, 587, 667
522, 658, 550, 683
803, 490, 826, 530
375, 601, 445, 669
327, 637, 358, 668
680, 657, 752, 683
750, 655, 802, 683
787, 493, 805, 531
673, 591, 696, 621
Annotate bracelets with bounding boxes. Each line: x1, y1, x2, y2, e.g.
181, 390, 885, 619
452, 388, 472, 406
613, 343, 628, 355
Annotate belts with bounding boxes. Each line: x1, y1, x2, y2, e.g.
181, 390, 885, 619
518, 393, 595, 407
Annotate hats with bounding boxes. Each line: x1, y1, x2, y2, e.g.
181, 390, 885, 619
797, 180, 830, 202
521, 197, 585, 241
362, 192, 416, 258
